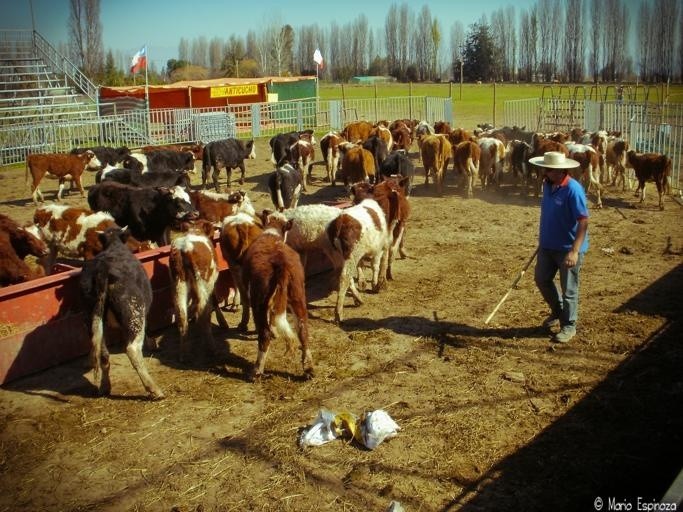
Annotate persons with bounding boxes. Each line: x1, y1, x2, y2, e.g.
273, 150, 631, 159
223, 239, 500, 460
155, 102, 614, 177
528, 151, 590, 343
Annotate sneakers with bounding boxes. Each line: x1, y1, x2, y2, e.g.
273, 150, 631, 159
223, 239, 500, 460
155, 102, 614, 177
541, 308, 564, 329
555, 323, 575, 344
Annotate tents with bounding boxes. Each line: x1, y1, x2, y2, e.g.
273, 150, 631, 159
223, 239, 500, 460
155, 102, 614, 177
98, 75, 318, 141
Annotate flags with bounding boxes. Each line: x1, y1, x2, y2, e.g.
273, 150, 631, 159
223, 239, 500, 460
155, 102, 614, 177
129, 44, 148, 75
313, 46, 323, 69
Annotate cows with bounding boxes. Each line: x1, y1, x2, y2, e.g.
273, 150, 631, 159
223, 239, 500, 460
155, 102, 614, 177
452, 141, 480, 197
416, 121, 435, 163
238, 210, 316, 383
0, 215, 50, 258
88, 182, 199, 246
123, 150, 196, 173
449, 128, 472, 145
201, 137, 257, 194
285, 204, 367, 290
402, 119, 420, 129
70, 145, 132, 184
474, 122, 631, 209
320, 131, 343, 186
434, 122, 450, 134
24, 151, 102, 207
326, 183, 387, 325
290, 130, 315, 191
374, 176, 411, 286
169, 221, 230, 365
83, 225, 164, 400
144, 141, 203, 172
338, 142, 375, 193
105, 169, 192, 192
341, 121, 373, 144
219, 211, 264, 313
417, 135, 452, 195
627, 150, 675, 211
269, 148, 304, 213
270, 131, 301, 162
189, 190, 256, 241
0, 231, 44, 287
390, 120, 411, 151
380, 150, 414, 198
34, 204, 159, 276
369, 120, 393, 154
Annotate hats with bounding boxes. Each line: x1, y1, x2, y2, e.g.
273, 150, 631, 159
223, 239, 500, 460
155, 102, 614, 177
526, 151, 581, 170
